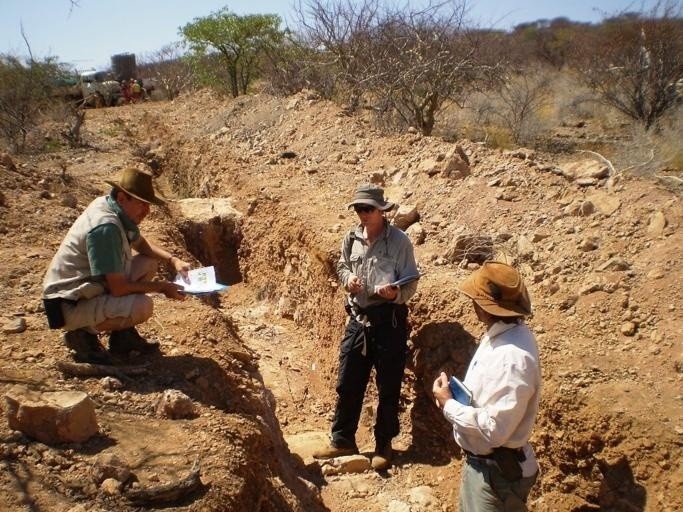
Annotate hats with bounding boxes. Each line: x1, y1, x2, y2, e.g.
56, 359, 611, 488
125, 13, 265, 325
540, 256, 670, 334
99, 166, 167, 208
346, 188, 398, 213
453, 259, 533, 319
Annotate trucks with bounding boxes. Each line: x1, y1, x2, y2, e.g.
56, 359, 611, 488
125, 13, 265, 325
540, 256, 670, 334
57, 70, 121, 108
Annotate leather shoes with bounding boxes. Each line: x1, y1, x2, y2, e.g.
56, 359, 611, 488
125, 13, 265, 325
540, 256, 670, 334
370, 440, 394, 471
310, 443, 360, 461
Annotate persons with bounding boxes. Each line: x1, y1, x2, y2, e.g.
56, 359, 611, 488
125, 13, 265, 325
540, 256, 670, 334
312, 182, 420, 470
431, 260, 542, 511
43, 168, 191, 364
121, 77, 141, 105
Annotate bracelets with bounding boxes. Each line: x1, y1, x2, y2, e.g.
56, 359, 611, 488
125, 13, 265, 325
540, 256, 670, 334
168, 254, 173, 264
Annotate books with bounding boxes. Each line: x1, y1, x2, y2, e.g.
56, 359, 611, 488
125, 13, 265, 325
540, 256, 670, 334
449, 374, 473, 406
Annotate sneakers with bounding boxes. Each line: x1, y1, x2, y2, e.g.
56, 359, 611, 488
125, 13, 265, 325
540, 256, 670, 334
61, 327, 117, 366
105, 325, 160, 356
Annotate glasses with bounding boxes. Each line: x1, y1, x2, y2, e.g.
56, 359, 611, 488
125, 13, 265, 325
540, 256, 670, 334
352, 205, 377, 214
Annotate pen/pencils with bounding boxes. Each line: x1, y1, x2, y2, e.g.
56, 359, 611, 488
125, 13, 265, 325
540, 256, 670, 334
472, 361, 477, 370
359, 284, 362, 287
